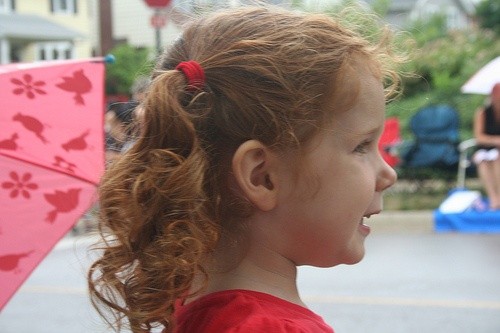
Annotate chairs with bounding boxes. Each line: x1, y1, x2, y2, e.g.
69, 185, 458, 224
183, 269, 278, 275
401, 102, 465, 189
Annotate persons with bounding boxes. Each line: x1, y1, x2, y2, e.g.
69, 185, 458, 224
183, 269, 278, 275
473, 83, 500, 210
125, 8, 397, 333
